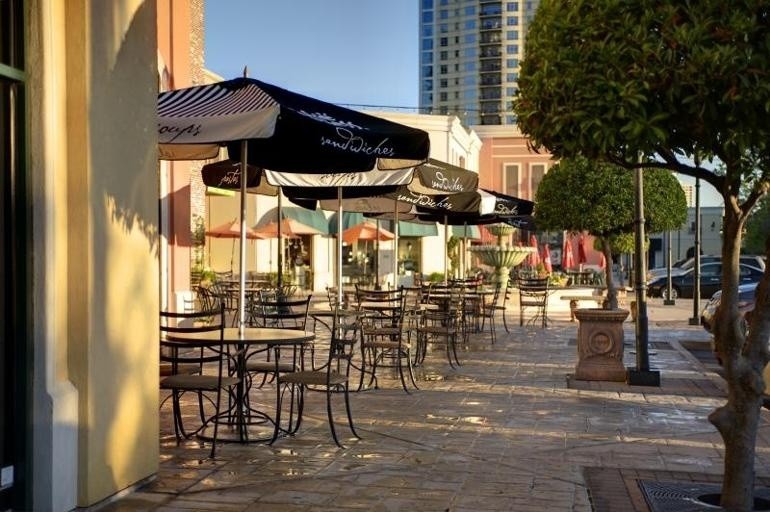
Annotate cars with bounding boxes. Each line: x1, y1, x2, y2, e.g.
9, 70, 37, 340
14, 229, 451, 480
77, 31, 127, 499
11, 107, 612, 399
646, 262, 763, 298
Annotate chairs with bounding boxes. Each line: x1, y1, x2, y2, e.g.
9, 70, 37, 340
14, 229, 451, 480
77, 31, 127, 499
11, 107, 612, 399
162, 303, 250, 460
520, 276, 551, 327
159, 270, 513, 395
265, 305, 367, 452
228, 294, 312, 432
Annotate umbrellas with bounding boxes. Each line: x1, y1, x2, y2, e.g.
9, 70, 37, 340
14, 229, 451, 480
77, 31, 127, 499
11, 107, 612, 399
200, 154, 481, 338
156, 65, 433, 339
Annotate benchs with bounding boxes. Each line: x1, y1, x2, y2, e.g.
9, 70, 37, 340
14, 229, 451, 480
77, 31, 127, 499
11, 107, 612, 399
560, 295, 627, 322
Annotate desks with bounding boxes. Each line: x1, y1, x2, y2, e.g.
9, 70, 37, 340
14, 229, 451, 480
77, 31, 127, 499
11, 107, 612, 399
165, 327, 315, 444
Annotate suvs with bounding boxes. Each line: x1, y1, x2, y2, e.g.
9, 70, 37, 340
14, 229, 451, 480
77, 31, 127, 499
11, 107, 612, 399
649, 254, 765, 280
700, 282, 759, 335
